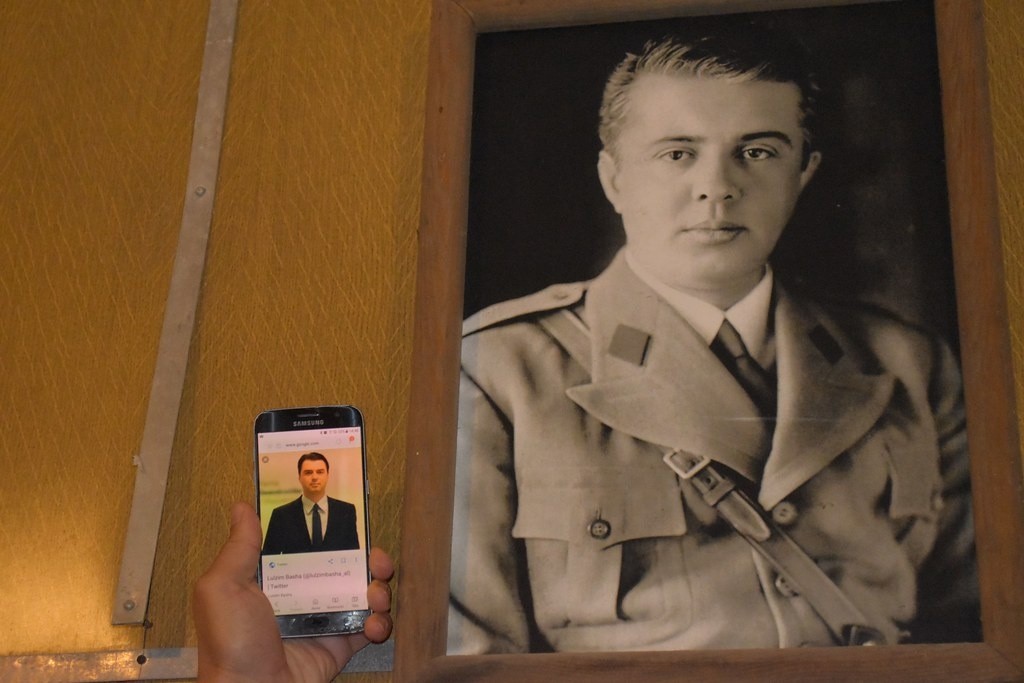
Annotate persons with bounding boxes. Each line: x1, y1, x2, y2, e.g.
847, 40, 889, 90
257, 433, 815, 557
262, 452, 360, 556
447, 30, 985, 656
192, 500, 394, 683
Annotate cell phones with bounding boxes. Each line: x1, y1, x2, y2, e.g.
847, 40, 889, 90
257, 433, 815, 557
253, 404, 374, 638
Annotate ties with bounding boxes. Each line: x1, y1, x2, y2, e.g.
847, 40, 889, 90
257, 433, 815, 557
312, 504, 323, 547
717, 320, 775, 409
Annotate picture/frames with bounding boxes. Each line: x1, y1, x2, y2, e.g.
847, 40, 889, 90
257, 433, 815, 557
392, 0, 1024, 682
